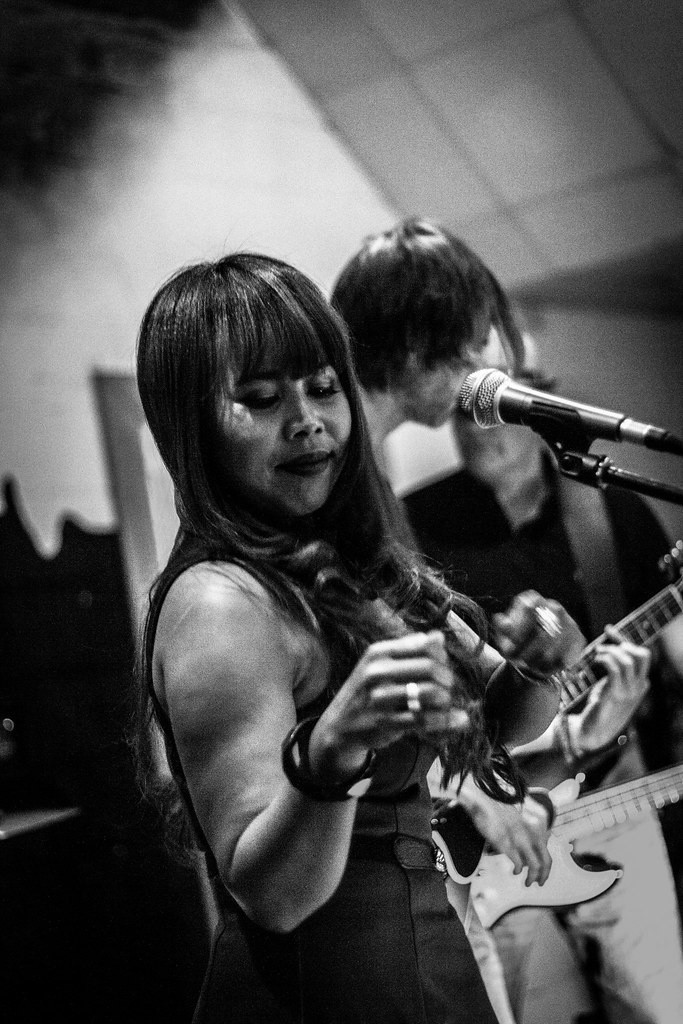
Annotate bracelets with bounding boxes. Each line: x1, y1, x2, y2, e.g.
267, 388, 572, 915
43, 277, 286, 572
279, 711, 378, 803
555, 710, 584, 766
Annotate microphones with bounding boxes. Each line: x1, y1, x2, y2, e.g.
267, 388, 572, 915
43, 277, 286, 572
459, 369, 683, 457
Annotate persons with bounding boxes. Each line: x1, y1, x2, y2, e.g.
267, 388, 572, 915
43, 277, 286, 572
322, 214, 654, 1023
129, 248, 593, 1022
396, 353, 683, 1023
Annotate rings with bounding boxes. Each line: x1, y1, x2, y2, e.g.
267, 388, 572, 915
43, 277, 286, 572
535, 605, 564, 640
407, 678, 422, 715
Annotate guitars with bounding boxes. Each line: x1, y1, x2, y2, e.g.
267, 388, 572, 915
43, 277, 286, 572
426, 752, 681, 930
422, 534, 682, 885
